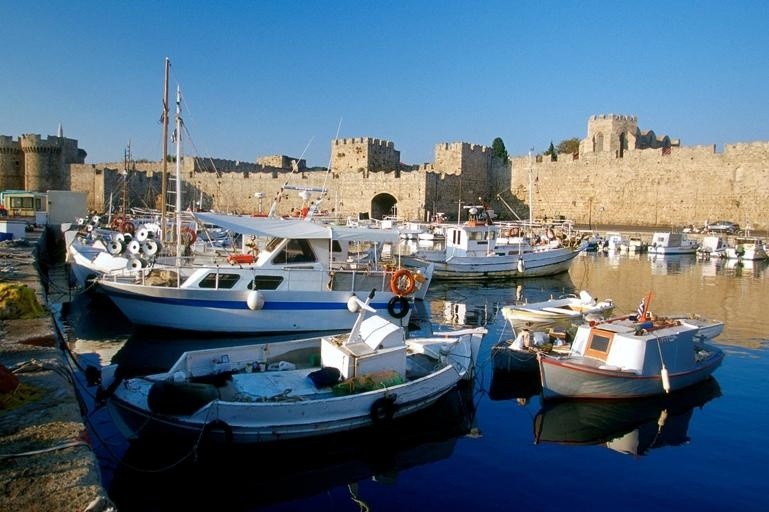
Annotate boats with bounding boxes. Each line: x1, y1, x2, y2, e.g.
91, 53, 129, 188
490, 337, 550, 374
574, 229, 768, 262
106, 289, 488, 443
536, 290, 725, 399
500, 290, 615, 341
668, 315, 725, 340
532, 376, 723, 457
1, 141, 589, 296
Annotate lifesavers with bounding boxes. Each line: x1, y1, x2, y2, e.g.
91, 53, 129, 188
392, 270, 414, 296
180, 227, 196, 244
114, 217, 135, 233
545, 229, 555, 240
228, 255, 252, 263
510, 228, 519, 237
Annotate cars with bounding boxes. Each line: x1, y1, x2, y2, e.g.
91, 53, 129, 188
704, 220, 740, 234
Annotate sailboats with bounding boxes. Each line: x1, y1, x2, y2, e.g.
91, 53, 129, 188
95, 56, 417, 333
